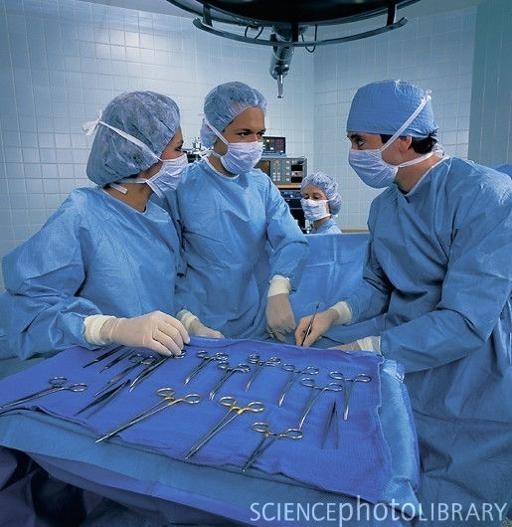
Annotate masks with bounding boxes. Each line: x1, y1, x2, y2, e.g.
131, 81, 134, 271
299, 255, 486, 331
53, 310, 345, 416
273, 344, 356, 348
220, 141, 265, 175
348, 149, 398, 187
145, 151, 189, 198
300, 197, 329, 221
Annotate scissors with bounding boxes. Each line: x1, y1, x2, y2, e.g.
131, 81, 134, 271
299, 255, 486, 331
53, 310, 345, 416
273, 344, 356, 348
0, 301, 372, 472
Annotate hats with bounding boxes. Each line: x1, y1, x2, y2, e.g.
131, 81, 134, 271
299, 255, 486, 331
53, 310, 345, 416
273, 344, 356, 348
86, 90, 180, 187
201, 81, 266, 146
346, 80, 440, 142
301, 169, 342, 214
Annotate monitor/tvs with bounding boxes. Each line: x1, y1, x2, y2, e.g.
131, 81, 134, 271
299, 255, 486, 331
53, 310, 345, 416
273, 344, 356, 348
262, 137, 286, 155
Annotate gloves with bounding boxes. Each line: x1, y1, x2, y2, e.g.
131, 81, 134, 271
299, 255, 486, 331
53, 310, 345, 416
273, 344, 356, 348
295, 298, 353, 348
177, 308, 226, 338
100, 310, 191, 356
329, 334, 382, 356
263, 277, 296, 342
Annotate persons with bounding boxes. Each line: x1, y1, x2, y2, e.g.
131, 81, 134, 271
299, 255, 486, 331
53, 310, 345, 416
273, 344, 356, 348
1, 89, 225, 361
148, 80, 308, 342
293, 78, 511, 525
299, 170, 346, 234
